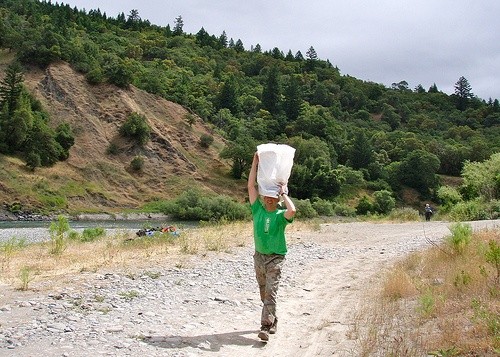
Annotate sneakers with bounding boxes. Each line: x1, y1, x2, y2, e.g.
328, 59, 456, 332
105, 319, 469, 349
258, 326, 269, 341
270, 319, 277, 334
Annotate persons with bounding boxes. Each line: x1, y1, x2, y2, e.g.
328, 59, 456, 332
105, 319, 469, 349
247, 151, 296, 344
425, 204, 433, 221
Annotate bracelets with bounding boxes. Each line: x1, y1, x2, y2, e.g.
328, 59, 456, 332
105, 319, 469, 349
281, 193, 286, 196
